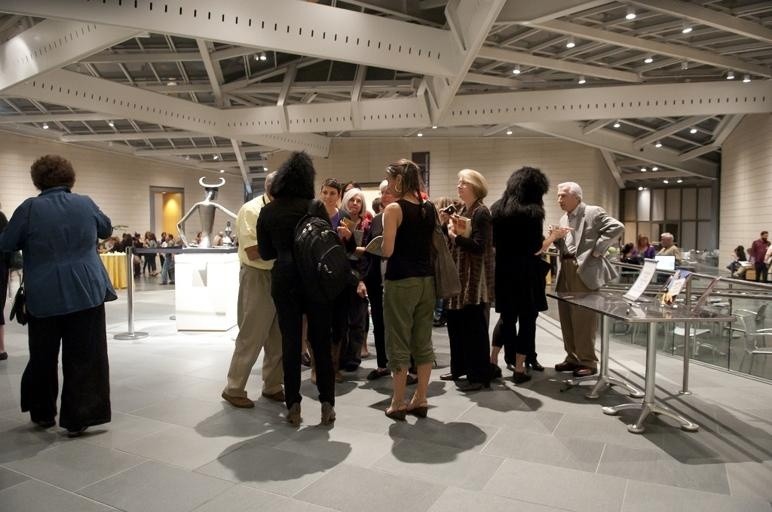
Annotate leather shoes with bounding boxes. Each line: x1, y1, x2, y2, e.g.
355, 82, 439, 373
572, 365, 598, 377
555, 361, 577, 372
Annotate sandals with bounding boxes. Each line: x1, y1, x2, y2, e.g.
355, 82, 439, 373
384, 399, 409, 423
406, 392, 429, 419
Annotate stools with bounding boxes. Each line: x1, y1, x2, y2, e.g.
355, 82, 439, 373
674, 327, 726, 360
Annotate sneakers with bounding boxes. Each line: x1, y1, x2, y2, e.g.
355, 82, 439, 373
0, 352, 8, 361
360, 349, 369, 358
300, 352, 312, 367
367, 368, 391, 380
159, 280, 175, 285
66, 424, 90, 438
406, 374, 418, 385
34, 417, 57, 429
513, 372, 531, 384
149, 271, 160, 276
262, 389, 285, 401
344, 357, 361, 372
222, 391, 255, 408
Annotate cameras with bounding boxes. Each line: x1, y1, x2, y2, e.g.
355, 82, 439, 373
444, 205, 457, 215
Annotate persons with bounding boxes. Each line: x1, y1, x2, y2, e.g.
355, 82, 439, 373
621, 240, 637, 260
631, 233, 656, 258
255, 150, 338, 425
0, 153, 118, 435
220, 171, 285, 409
0, 208, 13, 361
489, 166, 573, 383
656, 232, 681, 262
96, 231, 240, 286
439, 167, 493, 393
751, 231, 769, 281
379, 159, 435, 420
299, 176, 545, 385
727, 244, 772, 279
547, 180, 625, 376
177, 188, 238, 250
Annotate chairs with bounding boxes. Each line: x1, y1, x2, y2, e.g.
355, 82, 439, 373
739, 315, 772, 374
721, 302, 768, 358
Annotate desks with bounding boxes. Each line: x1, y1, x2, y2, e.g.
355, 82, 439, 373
100, 254, 133, 289
545, 290, 737, 434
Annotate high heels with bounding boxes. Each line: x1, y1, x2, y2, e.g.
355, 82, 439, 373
525, 359, 544, 371
439, 372, 454, 380
286, 402, 301, 430
505, 359, 516, 369
321, 400, 336, 426
459, 378, 491, 391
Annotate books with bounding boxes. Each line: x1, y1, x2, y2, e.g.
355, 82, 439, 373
622, 258, 661, 303
658, 269, 693, 307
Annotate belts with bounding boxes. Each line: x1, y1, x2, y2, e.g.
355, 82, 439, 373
562, 253, 576, 261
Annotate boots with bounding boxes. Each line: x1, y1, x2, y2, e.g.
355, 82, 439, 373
330, 340, 345, 383
310, 365, 318, 385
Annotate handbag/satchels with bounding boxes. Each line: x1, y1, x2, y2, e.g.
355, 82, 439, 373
427, 201, 463, 299
9, 287, 29, 325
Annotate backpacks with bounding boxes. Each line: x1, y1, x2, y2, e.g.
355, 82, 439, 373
290, 198, 353, 306
